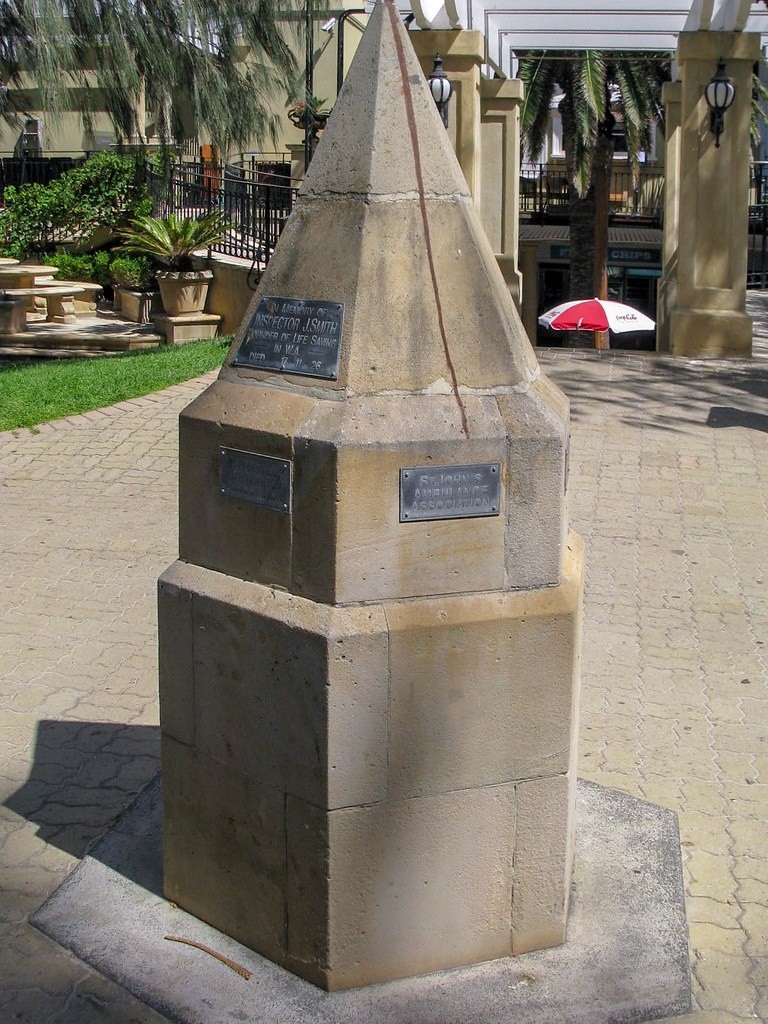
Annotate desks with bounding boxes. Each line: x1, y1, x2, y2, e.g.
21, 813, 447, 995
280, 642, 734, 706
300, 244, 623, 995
0, 265, 59, 312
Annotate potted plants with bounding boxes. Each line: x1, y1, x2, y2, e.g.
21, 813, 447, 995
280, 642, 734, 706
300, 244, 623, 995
109, 209, 242, 324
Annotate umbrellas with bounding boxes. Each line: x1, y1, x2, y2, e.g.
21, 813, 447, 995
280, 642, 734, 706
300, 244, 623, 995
536, 297, 657, 334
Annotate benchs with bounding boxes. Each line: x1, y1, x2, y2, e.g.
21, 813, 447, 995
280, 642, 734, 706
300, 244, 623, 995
0, 281, 103, 334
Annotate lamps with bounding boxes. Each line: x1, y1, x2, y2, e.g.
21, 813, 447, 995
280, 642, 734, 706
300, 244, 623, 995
705, 54, 737, 149
426, 52, 454, 130
